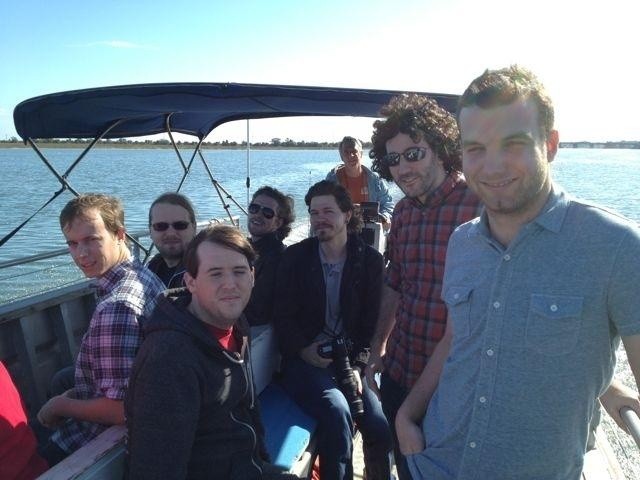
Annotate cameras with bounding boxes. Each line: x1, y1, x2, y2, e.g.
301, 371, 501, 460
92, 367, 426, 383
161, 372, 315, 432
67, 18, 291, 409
318, 336, 368, 418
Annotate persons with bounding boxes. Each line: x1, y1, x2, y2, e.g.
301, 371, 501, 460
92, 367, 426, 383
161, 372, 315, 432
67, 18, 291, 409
365, 93, 640, 480
243, 186, 295, 329
325, 136, 394, 230
272, 180, 391, 480
126, 225, 301, 480
395, 67, 640, 480
28, 192, 168, 470
146, 192, 197, 289
0, 362, 48, 480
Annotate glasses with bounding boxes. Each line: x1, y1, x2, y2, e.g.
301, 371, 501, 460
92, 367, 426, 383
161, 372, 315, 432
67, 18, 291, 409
385, 147, 425, 166
248, 204, 274, 219
150, 221, 188, 231
